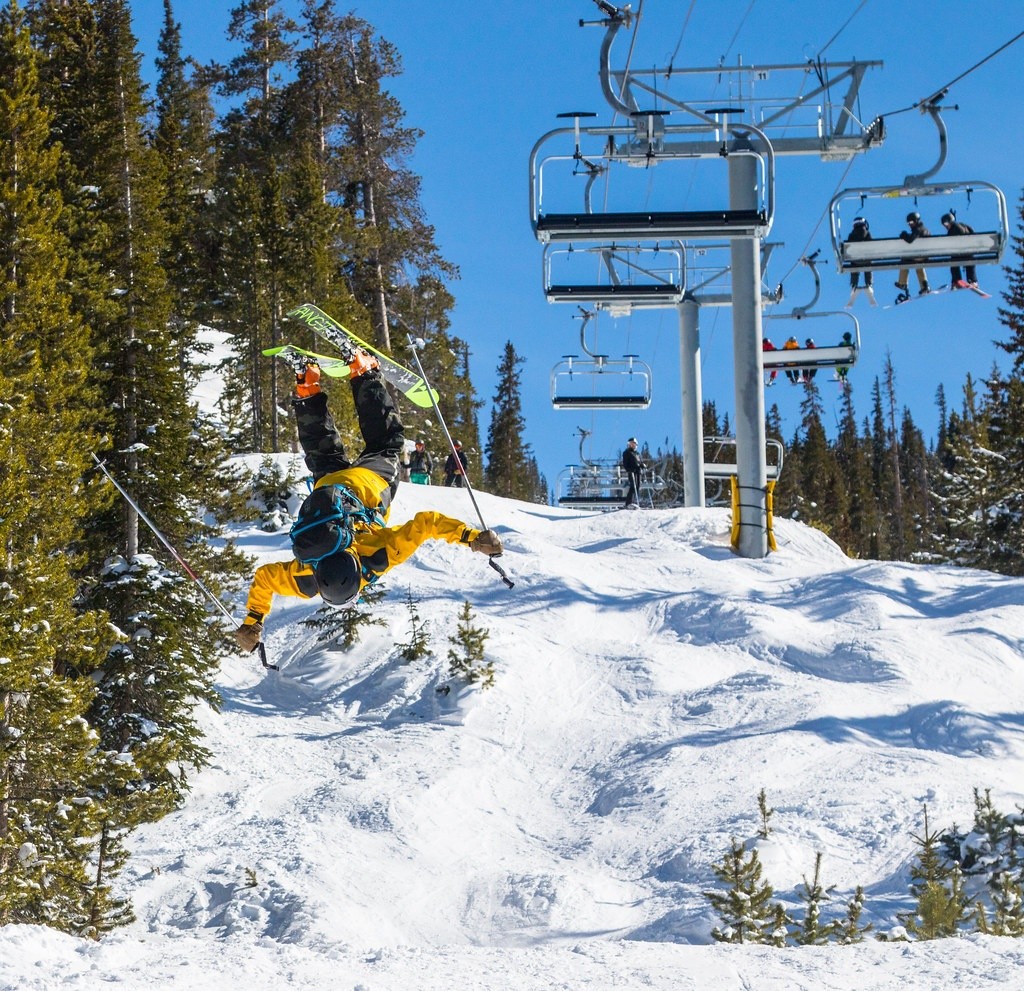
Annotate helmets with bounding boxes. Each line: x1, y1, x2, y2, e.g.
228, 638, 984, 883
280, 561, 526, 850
763, 338, 771, 343
453, 440, 462, 447
805, 338, 813, 345
789, 336, 796, 342
415, 440, 424, 445
843, 332, 852, 339
628, 438, 638, 445
852, 212, 955, 230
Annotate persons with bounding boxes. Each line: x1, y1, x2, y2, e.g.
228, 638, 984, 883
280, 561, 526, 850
893, 212, 931, 294
802, 338, 817, 383
834, 332, 855, 379
233, 347, 503, 652
400, 438, 433, 485
844, 216, 873, 297
762, 338, 777, 380
444, 440, 468, 488
622, 437, 647, 510
782, 336, 799, 382
941, 213, 978, 290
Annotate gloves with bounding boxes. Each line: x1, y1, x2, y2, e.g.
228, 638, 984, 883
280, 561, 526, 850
399, 460, 405, 468
232, 622, 264, 652
454, 469, 461, 475
470, 529, 503, 559
427, 470, 432, 475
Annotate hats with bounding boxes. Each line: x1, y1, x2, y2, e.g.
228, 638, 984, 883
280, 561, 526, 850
317, 551, 360, 609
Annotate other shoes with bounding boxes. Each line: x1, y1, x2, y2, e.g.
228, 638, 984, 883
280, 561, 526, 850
895, 282, 931, 294
850, 281, 873, 289
952, 279, 979, 288
626, 503, 638, 509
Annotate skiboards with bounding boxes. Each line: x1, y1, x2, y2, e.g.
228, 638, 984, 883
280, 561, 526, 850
285, 302, 440, 409
763, 377, 851, 387
842, 277, 993, 310
261, 344, 351, 378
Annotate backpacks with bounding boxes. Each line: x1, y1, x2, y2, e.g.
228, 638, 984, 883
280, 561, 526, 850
289, 484, 366, 563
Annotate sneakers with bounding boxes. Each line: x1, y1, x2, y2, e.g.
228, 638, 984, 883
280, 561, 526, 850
295, 364, 321, 397
349, 350, 381, 380
